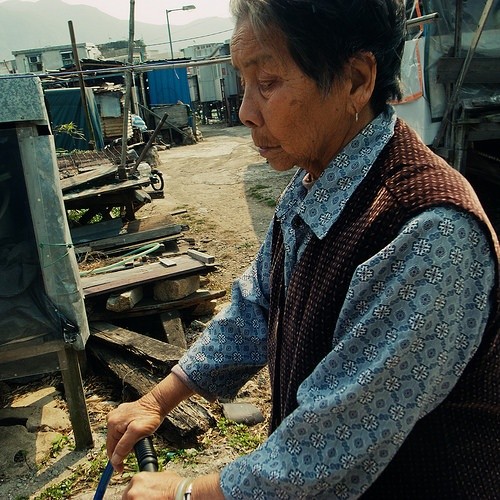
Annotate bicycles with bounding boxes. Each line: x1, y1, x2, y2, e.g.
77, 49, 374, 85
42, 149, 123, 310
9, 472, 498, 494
146, 161, 164, 191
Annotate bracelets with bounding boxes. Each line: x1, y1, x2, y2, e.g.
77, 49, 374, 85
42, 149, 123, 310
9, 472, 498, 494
174, 476, 194, 500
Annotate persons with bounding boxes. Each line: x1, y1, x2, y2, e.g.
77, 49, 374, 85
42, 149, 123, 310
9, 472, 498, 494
107, 0, 500, 500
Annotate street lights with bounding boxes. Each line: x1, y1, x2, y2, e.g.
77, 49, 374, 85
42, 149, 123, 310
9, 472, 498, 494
165, 5, 196, 60
